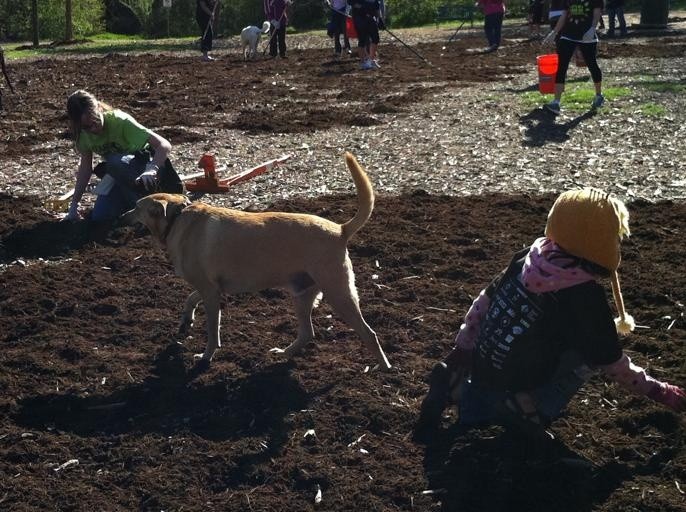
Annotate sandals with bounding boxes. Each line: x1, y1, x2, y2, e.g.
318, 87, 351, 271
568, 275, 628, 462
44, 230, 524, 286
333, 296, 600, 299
418, 363, 463, 432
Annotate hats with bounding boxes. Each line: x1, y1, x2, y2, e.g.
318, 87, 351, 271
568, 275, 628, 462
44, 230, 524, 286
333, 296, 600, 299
544, 183, 632, 276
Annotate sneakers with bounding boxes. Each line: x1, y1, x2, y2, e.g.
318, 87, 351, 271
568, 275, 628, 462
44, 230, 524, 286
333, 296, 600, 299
332, 47, 381, 70
596, 92, 604, 107
543, 102, 561, 116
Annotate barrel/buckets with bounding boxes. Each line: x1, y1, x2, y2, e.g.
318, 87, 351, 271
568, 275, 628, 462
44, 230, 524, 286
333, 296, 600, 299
575, 47, 588, 67
536, 54, 565, 94
345, 17, 358, 39
640, 0, 670, 29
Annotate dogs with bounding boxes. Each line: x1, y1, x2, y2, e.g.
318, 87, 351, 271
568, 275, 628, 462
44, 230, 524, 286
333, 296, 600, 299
241, 21, 271, 62
118, 151, 393, 373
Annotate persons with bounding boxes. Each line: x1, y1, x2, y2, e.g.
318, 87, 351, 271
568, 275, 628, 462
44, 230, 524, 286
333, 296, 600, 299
348, 1, 386, 71
196, 0, 215, 63
64, 88, 183, 224
526, 0, 627, 39
262, 0, 290, 60
536, 1, 604, 116
325, 1, 353, 57
474, 0, 506, 54
420, 187, 686, 468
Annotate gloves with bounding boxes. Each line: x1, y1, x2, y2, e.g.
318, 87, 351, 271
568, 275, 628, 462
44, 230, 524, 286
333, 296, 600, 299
377, 17, 386, 31
368, 15, 377, 24
543, 30, 559, 44
582, 26, 596, 43
284, 0, 293, 7
67, 202, 85, 224
135, 163, 161, 192
271, 18, 280, 30
